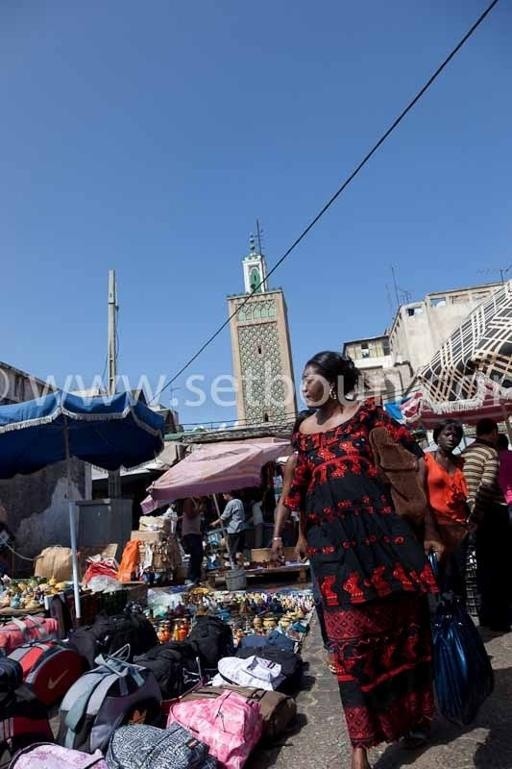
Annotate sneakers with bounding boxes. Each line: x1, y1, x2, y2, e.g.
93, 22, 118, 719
326, 653, 337, 674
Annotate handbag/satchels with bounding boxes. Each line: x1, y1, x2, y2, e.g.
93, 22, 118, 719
428, 593, 494, 726
0, 614, 236, 768
433, 524, 477, 545
370, 427, 427, 517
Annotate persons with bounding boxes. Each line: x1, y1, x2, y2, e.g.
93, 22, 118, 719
159, 477, 297, 590
267, 350, 449, 768
413, 414, 512, 635
293, 510, 340, 675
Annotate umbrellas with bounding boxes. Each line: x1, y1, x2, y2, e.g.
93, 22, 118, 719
0, 388, 167, 624
137, 436, 295, 568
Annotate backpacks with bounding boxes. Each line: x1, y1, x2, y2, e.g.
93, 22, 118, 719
209, 655, 287, 690
232, 629, 302, 674
